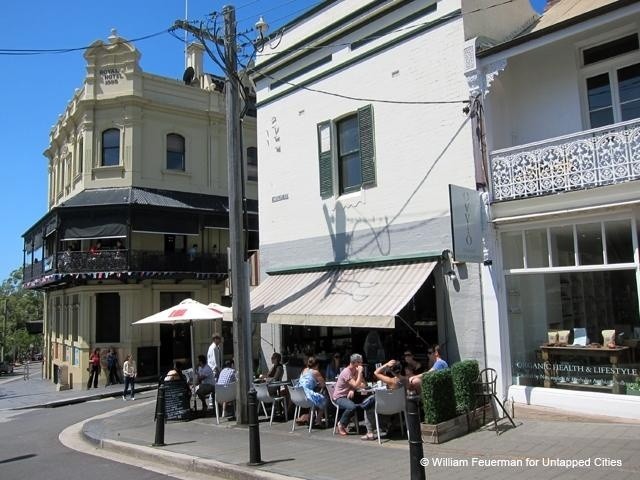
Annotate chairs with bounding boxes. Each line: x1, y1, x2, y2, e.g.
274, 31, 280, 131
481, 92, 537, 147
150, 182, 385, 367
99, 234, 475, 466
191, 367, 407, 448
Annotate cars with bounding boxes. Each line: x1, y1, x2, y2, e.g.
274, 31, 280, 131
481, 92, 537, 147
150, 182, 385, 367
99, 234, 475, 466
0, 361, 13, 374
30, 352, 43, 360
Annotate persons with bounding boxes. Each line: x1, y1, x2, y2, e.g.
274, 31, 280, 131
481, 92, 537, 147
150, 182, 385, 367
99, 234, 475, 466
187, 243, 199, 263
163, 361, 186, 381
61, 245, 77, 273
114, 240, 130, 270
85, 242, 104, 271
86, 347, 102, 390
122, 354, 138, 402
107, 345, 123, 385
195, 332, 449, 443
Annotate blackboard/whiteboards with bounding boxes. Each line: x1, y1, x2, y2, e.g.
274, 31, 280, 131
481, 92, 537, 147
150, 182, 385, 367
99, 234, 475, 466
158, 378, 191, 422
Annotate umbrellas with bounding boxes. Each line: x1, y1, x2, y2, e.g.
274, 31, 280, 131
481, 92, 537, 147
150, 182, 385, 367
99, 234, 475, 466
204, 302, 230, 332
125, 296, 231, 418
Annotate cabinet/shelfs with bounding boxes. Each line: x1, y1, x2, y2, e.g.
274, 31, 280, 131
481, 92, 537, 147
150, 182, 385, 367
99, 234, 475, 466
540, 348, 628, 395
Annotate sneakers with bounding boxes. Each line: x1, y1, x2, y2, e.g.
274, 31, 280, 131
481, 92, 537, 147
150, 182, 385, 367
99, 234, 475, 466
336, 422, 347, 435
361, 433, 375, 440
